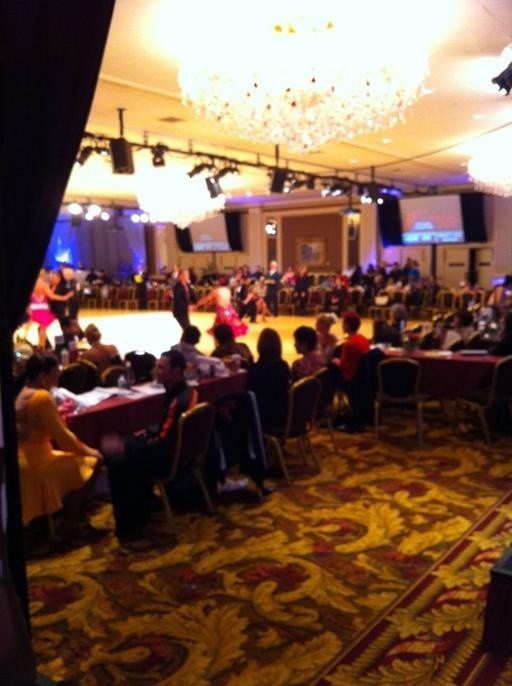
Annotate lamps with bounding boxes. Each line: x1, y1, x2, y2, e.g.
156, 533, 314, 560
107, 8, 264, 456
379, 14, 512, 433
73, 101, 386, 213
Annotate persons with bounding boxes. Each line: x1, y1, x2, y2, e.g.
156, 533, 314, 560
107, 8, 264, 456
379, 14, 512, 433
290, 255, 510, 436
11, 256, 290, 534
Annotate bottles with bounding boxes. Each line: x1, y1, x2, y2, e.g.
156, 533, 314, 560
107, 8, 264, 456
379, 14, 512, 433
118, 374, 127, 391
61, 348, 70, 366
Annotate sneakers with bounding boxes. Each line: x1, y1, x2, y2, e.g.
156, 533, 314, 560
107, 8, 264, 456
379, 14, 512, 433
217, 476, 248, 496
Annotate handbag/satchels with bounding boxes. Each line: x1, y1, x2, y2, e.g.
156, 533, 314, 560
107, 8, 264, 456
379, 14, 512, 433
124, 347, 156, 383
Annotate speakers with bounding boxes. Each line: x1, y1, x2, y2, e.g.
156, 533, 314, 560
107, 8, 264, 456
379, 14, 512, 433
109, 138, 135, 174
367, 181, 378, 197
267, 169, 286, 192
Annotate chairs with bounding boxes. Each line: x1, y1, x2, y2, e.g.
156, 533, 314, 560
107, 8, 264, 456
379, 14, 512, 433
327, 325, 510, 448
16, 342, 326, 543
80, 265, 510, 322
